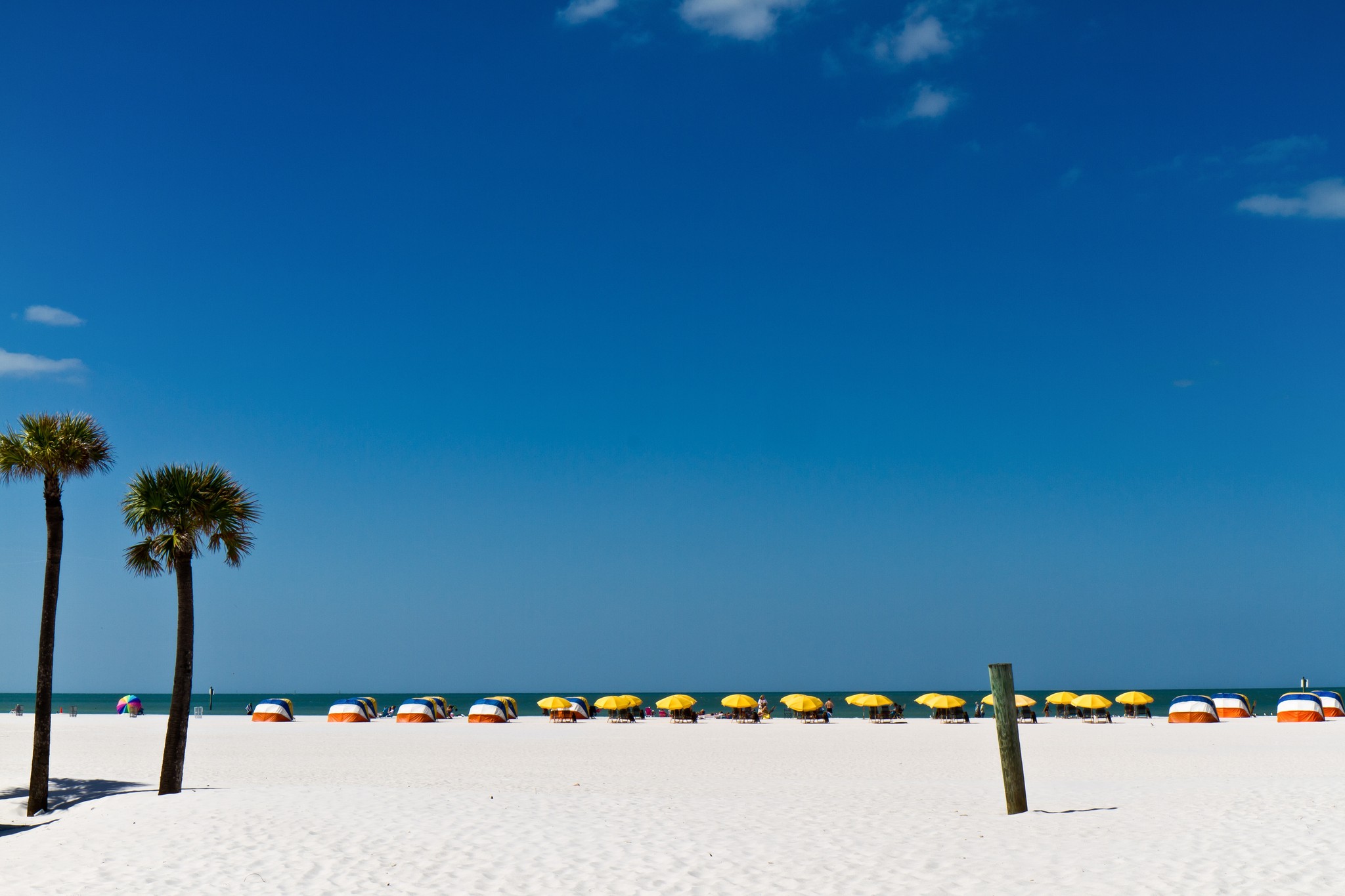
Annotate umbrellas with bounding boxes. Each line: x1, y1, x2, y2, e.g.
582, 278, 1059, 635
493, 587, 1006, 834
1045, 691, 1079, 719
1071, 694, 1113, 722
655, 694, 697, 723
981, 693, 1037, 707
537, 696, 572, 723
780, 694, 807, 719
594, 695, 631, 722
857, 694, 894, 723
926, 695, 966, 723
618, 695, 643, 707
845, 693, 873, 719
721, 694, 758, 723
116, 695, 141, 715
914, 693, 943, 719
1115, 691, 1154, 718
785, 696, 824, 723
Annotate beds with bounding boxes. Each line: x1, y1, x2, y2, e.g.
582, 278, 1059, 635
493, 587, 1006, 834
716, 714, 732, 719
697, 714, 705, 719
705, 713, 724, 719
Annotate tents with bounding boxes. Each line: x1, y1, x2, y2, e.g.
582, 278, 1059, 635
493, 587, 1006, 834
1209, 692, 1252, 719
252, 698, 296, 723
1309, 690, 1345, 717
1277, 692, 1327, 723
468, 697, 510, 723
1168, 694, 1220, 722
328, 699, 371, 723
550, 696, 590, 719
349, 696, 378, 719
494, 696, 518, 720
423, 696, 449, 719
396, 697, 438, 723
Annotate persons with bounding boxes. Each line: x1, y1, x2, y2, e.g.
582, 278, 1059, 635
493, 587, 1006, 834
1042, 701, 1052, 717
588, 705, 734, 718
824, 697, 834, 718
377, 705, 397, 718
446, 705, 452, 715
9, 704, 19, 713
889, 702, 902, 719
745, 695, 769, 723
245, 702, 253, 716
980, 701, 985, 718
140, 704, 144, 715
975, 701, 980, 718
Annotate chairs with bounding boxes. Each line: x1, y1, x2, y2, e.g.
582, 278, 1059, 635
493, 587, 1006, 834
1017, 706, 1038, 724
784, 709, 793, 719
868, 706, 894, 723
1137, 704, 1148, 719
607, 710, 621, 723
387, 708, 396, 717
731, 711, 761, 724
893, 704, 906, 719
378, 707, 389, 718
446, 705, 455, 718
549, 706, 577, 723
1082, 707, 1096, 723
645, 707, 655, 718
1066, 703, 1078, 718
1124, 703, 1135, 719
1095, 707, 1109, 723
589, 705, 596, 720
1055, 704, 1067, 718
659, 710, 666, 717
618, 707, 645, 723
796, 710, 830, 724
938, 707, 970, 723
670, 707, 698, 723
760, 706, 777, 719
